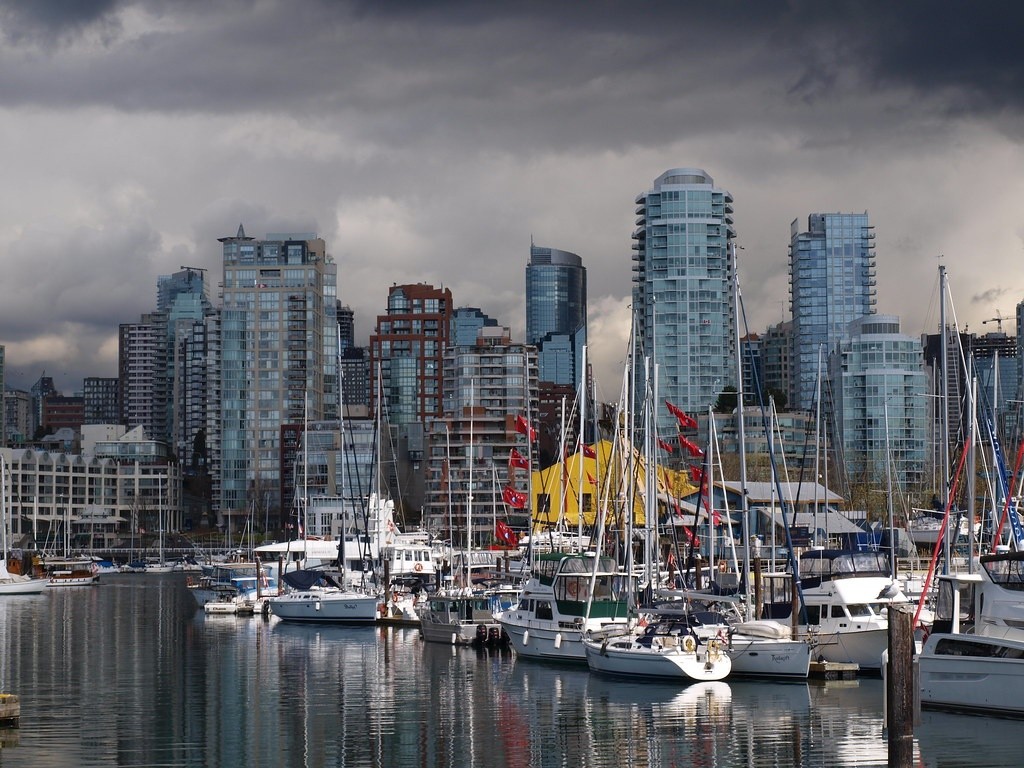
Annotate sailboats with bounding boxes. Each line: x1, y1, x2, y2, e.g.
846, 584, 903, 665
0, 242, 1022, 724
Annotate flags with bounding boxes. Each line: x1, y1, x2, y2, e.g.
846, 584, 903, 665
674, 498, 684, 519
262, 571, 268, 589
689, 463, 709, 483
514, 413, 536, 443
586, 471, 597, 486
502, 484, 528, 509
496, 520, 519, 550
664, 470, 672, 489
665, 400, 698, 428
583, 444, 597, 459
509, 449, 529, 470
678, 434, 704, 457
701, 498, 722, 527
683, 525, 699, 547
659, 439, 672, 453
702, 484, 708, 496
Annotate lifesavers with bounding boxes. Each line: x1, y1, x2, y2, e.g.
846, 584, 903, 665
415, 563, 423, 572
718, 562, 726, 572
392, 594, 398, 602
667, 582, 674, 589
682, 636, 696, 651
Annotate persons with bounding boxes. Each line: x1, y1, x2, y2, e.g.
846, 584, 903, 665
750, 535, 761, 559
